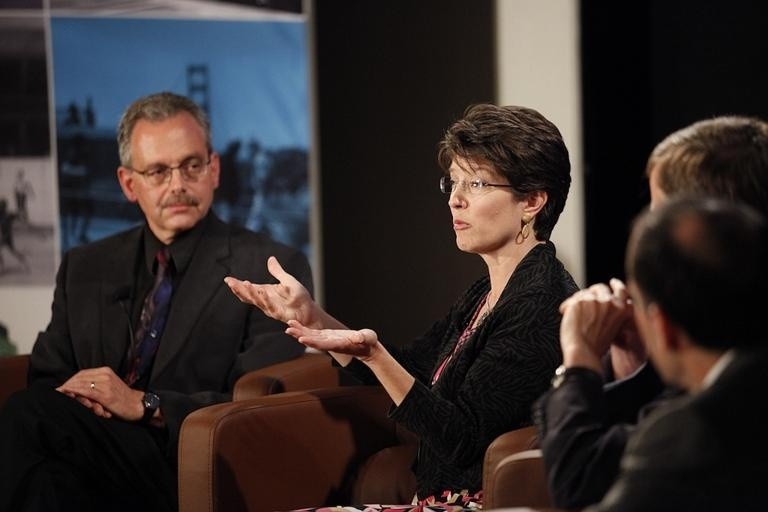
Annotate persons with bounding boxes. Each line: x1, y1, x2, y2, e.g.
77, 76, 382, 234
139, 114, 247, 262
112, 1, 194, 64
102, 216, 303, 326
1, 168, 35, 270
0, 88, 311, 510
528, 113, 767, 510
583, 195, 768, 512
221, 138, 278, 234
224, 102, 582, 512
66, 100, 104, 243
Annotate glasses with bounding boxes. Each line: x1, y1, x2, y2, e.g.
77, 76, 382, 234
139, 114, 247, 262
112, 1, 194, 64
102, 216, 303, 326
124, 153, 212, 187
439, 175, 521, 194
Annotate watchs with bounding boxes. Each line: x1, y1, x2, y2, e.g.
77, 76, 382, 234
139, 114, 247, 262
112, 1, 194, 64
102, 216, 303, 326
139, 389, 161, 424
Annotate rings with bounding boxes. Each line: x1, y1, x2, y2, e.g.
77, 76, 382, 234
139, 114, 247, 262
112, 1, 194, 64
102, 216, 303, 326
89, 381, 96, 390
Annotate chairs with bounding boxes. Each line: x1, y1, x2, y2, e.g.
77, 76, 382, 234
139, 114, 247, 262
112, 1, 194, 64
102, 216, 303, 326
2, 348, 554, 511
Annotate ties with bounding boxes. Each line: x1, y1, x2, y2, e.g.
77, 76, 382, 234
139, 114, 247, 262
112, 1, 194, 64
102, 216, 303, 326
123, 248, 173, 389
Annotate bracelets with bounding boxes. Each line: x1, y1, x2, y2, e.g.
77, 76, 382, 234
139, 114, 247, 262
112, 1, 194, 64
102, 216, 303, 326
550, 364, 602, 391
